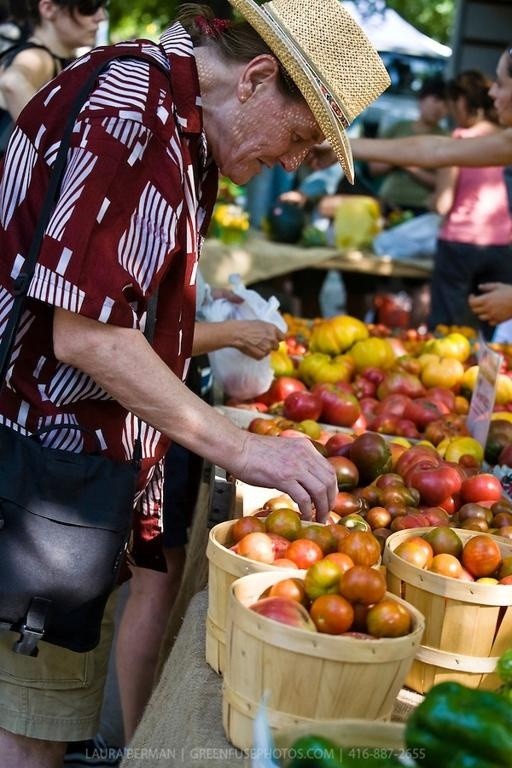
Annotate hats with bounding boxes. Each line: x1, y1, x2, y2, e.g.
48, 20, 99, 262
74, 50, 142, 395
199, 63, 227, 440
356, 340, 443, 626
228, 0, 393, 187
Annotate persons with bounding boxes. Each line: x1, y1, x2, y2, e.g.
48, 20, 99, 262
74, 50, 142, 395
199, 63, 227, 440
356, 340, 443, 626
0, 0, 512, 768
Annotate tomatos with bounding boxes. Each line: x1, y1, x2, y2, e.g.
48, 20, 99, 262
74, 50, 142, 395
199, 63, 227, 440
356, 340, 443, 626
226, 366, 512, 641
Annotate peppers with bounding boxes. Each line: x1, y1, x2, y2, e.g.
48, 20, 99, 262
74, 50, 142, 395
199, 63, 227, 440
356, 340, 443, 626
285, 648, 512, 768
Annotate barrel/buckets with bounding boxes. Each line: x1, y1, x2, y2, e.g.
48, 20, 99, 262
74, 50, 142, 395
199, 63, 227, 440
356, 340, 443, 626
221, 570, 424, 758
204, 508, 384, 676
386, 522, 512, 698
260, 720, 418, 767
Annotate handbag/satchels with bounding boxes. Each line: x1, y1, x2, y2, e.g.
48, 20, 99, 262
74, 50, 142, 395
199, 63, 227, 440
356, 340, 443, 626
0, 424, 141, 658
